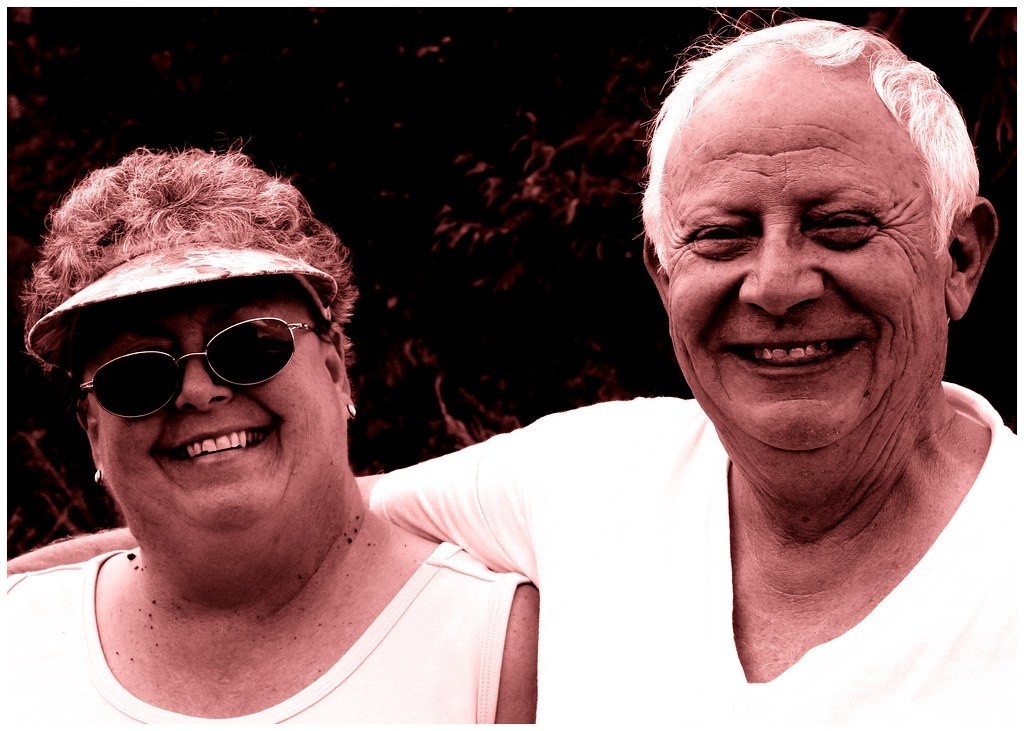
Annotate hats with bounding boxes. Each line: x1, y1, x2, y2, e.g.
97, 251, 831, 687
26, 227, 339, 374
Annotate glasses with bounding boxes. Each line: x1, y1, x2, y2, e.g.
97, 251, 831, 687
77, 316, 326, 418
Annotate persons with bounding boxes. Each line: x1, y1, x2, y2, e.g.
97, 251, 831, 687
6, 20, 1017, 731
6, 151, 539, 731
